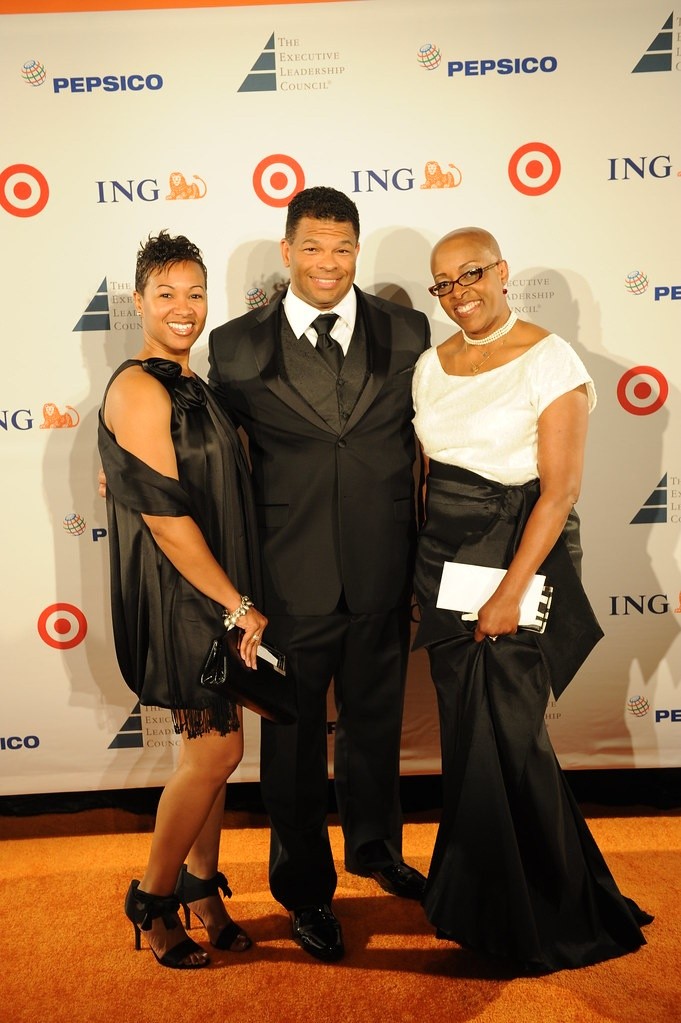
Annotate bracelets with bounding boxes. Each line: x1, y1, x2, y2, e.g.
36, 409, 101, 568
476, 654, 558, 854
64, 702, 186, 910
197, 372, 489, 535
221, 593, 255, 632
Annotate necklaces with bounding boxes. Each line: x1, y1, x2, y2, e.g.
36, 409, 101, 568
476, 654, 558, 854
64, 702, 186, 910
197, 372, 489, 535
461, 308, 518, 346
464, 334, 508, 376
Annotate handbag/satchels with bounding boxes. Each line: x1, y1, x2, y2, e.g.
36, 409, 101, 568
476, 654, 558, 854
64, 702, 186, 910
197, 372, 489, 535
199, 620, 296, 724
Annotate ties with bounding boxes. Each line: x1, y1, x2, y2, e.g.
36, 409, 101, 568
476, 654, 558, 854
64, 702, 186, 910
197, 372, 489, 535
308, 313, 345, 377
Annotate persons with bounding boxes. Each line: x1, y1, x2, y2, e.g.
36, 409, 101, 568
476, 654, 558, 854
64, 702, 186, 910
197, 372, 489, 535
96, 229, 270, 969
99, 186, 433, 966
406, 226, 654, 978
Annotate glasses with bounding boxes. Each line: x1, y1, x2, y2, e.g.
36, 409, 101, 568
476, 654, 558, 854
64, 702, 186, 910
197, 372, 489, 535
429, 262, 500, 297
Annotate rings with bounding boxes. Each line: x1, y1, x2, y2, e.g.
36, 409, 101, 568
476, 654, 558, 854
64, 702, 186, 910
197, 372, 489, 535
489, 636, 498, 642
253, 635, 259, 640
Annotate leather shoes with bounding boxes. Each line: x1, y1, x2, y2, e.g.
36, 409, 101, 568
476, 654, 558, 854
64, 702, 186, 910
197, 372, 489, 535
288, 900, 344, 964
345, 862, 428, 901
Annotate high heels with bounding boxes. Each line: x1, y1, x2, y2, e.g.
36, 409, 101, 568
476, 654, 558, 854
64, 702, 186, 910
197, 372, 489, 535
124, 878, 212, 969
176, 863, 253, 952
